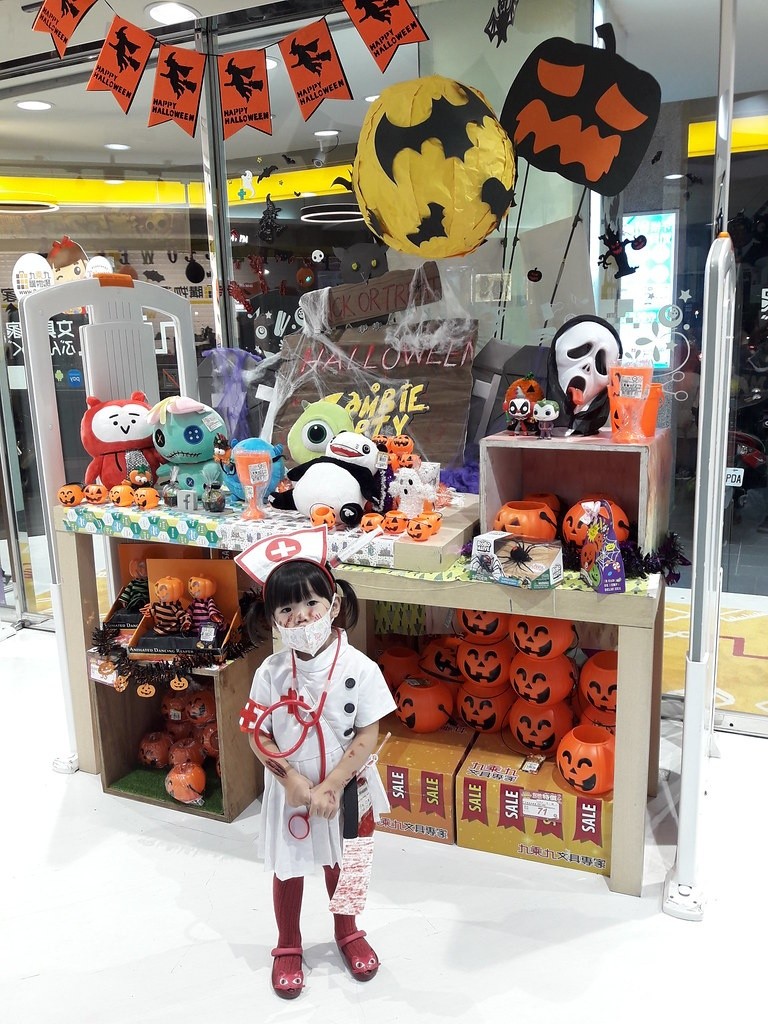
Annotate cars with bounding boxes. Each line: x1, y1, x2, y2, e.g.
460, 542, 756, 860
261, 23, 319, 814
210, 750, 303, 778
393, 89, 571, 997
729, 388, 767, 484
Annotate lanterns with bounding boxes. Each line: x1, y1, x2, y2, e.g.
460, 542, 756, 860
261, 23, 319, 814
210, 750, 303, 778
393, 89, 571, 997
309, 434, 630, 795
58, 482, 221, 802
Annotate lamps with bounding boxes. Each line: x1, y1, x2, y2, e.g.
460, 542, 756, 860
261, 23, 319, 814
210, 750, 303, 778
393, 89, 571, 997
299, 198, 364, 224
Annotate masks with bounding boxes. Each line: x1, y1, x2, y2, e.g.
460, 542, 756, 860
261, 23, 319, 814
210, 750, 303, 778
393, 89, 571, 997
273, 591, 336, 658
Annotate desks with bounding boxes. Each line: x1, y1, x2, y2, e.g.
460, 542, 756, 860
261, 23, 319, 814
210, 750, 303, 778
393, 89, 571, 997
52, 490, 667, 896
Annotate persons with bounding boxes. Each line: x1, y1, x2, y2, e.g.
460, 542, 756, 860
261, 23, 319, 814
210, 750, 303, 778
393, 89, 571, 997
234, 524, 398, 1000
533, 398, 559, 440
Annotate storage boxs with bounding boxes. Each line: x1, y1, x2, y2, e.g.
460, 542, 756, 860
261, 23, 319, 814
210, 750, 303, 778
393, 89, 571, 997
126, 558, 244, 663
277, 318, 481, 467
469, 530, 564, 589
454, 729, 613, 877
84, 621, 274, 822
481, 426, 673, 558
372, 714, 478, 847
578, 499, 626, 594
297, 260, 444, 327
100, 542, 204, 636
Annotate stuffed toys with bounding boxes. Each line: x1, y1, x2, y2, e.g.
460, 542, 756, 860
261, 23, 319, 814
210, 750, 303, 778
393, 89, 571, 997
80, 389, 387, 526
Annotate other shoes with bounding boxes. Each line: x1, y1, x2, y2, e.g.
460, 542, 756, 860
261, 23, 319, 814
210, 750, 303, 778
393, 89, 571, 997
334, 929, 382, 982
271, 944, 306, 1000
733, 514, 743, 525
757, 515, 768, 533
675, 469, 692, 481
